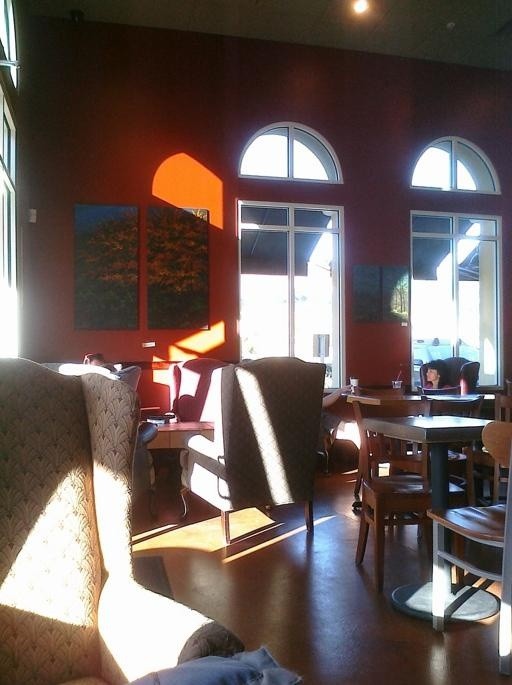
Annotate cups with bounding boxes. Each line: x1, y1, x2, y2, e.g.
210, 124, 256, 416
350, 377, 359, 392
392, 380, 402, 388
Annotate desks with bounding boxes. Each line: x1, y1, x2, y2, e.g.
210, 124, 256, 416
346, 394, 504, 406
362, 415, 501, 622
138, 423, 213, 449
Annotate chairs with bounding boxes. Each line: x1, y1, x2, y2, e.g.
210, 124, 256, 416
416, 357, 480, 395
352, 398, 466, 594
168, 357, 224, 423
179, 356, 328, 544
426, 423, 512, 574
40, 362, 160, 525
422, 396, 485, 419
0, 357, 244, 685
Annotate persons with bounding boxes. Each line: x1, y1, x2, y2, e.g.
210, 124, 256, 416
85, 354, 157, 502
318, 384, 358, 474
421, 359, 452, 390
